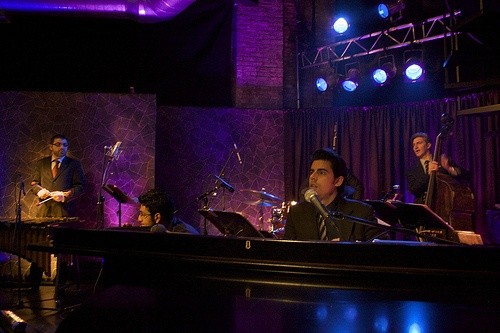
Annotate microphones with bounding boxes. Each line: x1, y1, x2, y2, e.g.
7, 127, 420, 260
214, 175, 234, 193
304, 189, 329, 219
260, 192, 282, 201
36, 198, 52, 206
108, 142, 122, 162
381, 185, 400, 201
234, 144, 242, 164
19, 174, 26, 195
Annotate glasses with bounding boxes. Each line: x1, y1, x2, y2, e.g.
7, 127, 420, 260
52, 142, 68, 147
138, 211, 154, 217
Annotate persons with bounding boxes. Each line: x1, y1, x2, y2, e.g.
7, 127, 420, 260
29, 134, 88, 217
283, 148, 379, 243
137, 190, 199, 234
406, 132, 465, 205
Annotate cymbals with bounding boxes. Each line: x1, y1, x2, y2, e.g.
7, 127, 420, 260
242, 200, 277, 206
240, 189, 285, 202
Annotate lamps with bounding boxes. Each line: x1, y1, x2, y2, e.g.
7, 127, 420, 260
342, 68, 360, 92
316, 62, 336, 92
372, 62, 397, 86
376, 0, 405, 20
402, 57, 426, 85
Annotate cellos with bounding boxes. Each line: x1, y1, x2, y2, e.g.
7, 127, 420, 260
417, 113, 483, 246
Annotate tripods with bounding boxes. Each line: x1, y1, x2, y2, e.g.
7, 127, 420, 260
0, 190, 65, 315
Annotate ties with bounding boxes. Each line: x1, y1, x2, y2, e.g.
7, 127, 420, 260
318, 214, 327, 240
422, 161, 429, 203
52, 161, 60, 179
